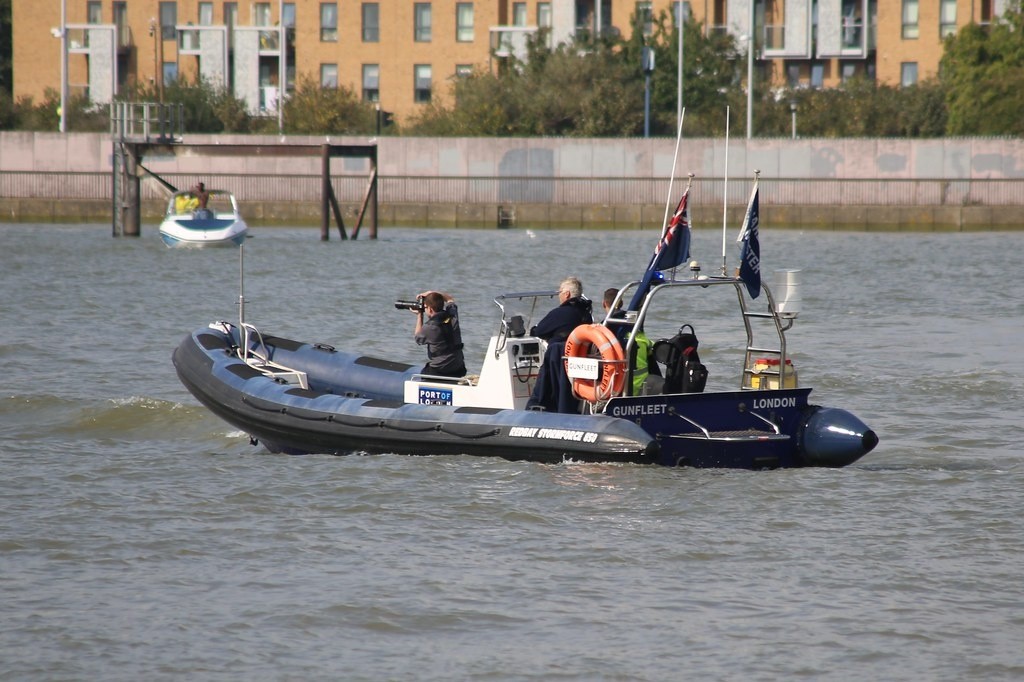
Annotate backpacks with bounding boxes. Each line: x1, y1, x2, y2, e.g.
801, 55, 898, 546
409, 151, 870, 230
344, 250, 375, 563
683, 359, 708, 393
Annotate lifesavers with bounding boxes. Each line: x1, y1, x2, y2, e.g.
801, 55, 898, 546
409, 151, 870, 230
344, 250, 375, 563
574, 321, 628, 400
562, 323, 619, 402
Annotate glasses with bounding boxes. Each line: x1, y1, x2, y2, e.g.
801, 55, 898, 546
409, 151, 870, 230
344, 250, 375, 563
423, 305, 427, 309
556, 290, 563, 293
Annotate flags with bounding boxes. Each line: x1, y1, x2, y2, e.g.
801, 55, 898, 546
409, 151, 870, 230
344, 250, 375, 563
738, 181, 761, 299
627, 193, 691, 310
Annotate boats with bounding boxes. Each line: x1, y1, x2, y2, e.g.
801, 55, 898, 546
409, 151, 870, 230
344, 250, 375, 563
171, 107, 878, 470
160, 188, 247, 249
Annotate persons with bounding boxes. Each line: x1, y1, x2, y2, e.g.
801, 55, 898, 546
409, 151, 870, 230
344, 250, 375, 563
409, 291, 467, 384
190, 183, 208, 208
624, 320, 663, 396
525, 278, 593, 414
588, 288, 639, 398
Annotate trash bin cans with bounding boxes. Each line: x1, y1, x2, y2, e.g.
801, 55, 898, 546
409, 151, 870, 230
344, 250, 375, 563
751, 357, 796, 390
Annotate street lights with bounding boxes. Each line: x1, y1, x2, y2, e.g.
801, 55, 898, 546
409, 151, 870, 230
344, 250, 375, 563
149, 18, 158, 99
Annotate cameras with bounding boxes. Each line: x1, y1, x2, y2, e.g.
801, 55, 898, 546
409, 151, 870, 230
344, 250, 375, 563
394, 296, 425, 313
